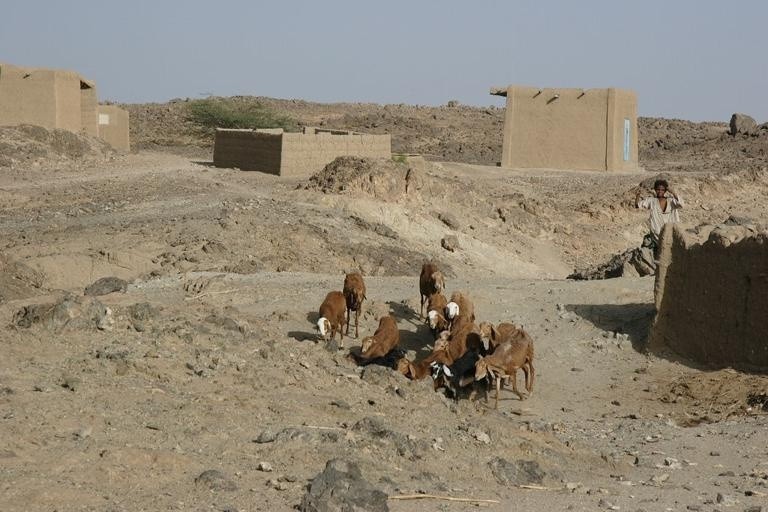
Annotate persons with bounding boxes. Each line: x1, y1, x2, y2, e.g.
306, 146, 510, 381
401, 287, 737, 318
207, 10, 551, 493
634, 179, 685, 249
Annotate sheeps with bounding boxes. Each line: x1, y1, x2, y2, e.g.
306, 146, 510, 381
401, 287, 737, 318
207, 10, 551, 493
342, 272, 367, 338
316, 290, 347, 351
397, 291, 535, 411
358, 316, 405, 371
419, 262, 447, 318
